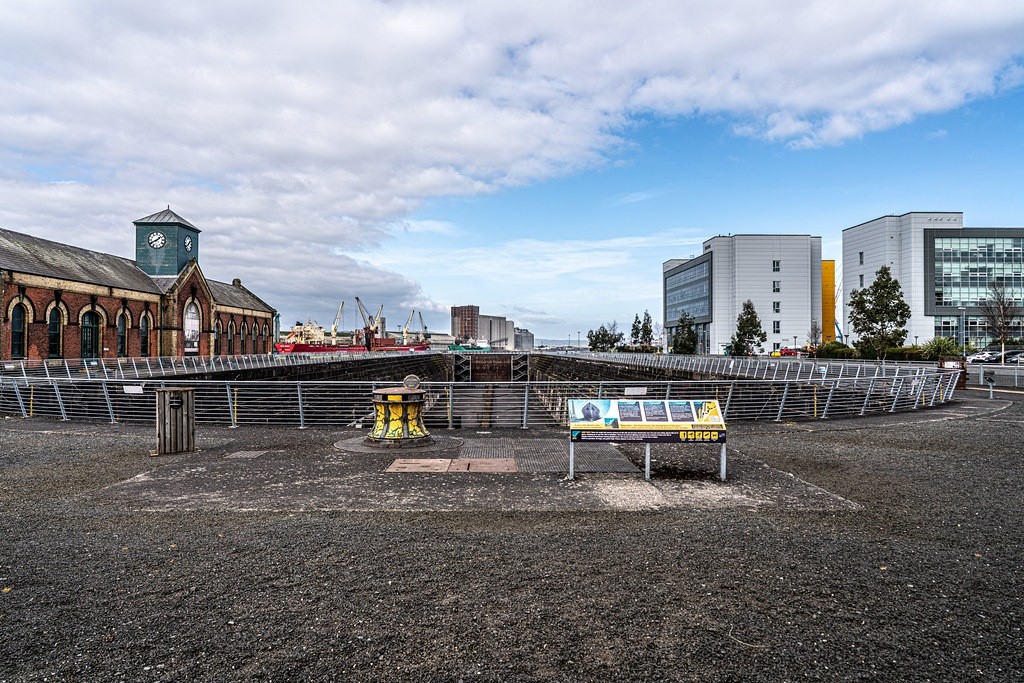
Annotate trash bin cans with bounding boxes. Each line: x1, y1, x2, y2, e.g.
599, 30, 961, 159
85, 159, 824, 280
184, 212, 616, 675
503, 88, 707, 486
938, 356, 966, 390
155, 387, 197, 453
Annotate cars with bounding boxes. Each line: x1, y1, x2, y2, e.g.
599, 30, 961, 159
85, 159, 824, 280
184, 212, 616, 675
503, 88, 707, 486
780, 347, 795, 356
966, 350, 1024, 365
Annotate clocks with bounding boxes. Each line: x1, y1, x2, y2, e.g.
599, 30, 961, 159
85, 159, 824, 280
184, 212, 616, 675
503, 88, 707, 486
147, 231, 166, 249
184, 235, 193, 253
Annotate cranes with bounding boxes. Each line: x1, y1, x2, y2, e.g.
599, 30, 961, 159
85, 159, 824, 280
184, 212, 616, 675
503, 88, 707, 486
402, 308, 416, 344
329, 301, 344, 337
370, 305, 385, 332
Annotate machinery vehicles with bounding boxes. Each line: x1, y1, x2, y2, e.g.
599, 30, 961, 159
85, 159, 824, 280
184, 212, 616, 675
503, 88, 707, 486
353, 295, 398, 346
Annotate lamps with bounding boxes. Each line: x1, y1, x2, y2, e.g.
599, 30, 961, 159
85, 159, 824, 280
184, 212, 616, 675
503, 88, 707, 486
983, 370, 996, 384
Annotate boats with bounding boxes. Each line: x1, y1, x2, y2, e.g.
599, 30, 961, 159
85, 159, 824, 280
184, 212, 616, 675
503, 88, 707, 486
273, 317, 432, 353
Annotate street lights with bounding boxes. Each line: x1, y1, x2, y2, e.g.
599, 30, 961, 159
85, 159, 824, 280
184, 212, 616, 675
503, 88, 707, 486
958, 307, 967, 356
826, 335, 831, 343
577, 331, 581, 350
844, 334, 849, 357
914, 336, 918, 347
793, 336, 798, 356
